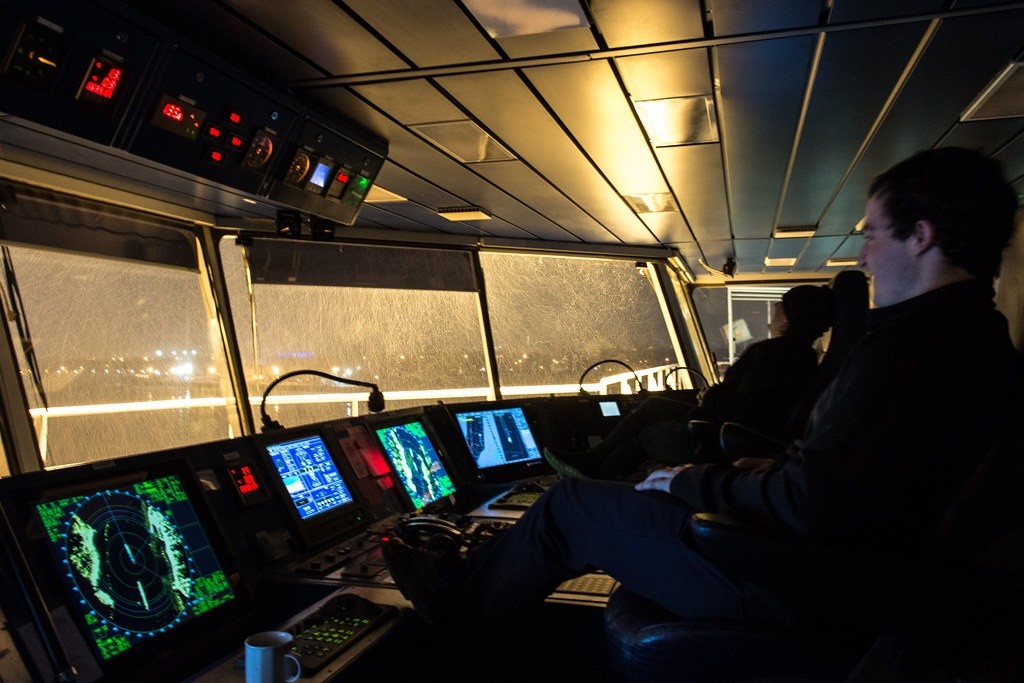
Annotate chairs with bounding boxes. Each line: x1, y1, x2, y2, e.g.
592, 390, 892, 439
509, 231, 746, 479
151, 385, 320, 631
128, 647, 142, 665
689, 270, 869, 459
602, 417, 1024, 683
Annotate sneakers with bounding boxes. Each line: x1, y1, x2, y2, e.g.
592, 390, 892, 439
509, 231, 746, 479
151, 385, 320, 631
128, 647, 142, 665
378, 534, 465, 628
542, 446, 613, 481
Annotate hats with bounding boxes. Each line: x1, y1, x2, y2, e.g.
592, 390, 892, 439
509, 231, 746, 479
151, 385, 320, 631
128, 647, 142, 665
782, 286, 836, 336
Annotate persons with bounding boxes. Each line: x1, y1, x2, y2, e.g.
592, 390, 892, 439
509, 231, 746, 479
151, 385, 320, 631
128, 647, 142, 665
380, 147, 1024, 653
543, 285, 832, 481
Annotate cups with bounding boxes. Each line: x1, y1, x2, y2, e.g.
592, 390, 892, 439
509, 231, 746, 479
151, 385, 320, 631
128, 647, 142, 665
244, 631, 301, 683
